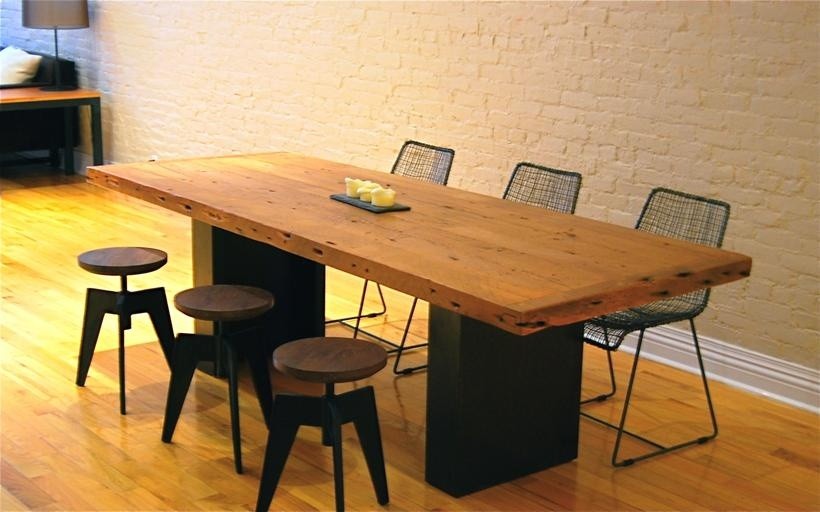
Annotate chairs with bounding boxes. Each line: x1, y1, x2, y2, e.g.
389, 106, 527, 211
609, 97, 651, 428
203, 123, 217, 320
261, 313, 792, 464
578, 190, 729, 465
327, 143, 454, 357
394, 163, 583, 375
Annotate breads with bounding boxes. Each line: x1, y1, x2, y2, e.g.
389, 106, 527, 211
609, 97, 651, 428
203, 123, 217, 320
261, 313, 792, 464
345, 178, 395, 207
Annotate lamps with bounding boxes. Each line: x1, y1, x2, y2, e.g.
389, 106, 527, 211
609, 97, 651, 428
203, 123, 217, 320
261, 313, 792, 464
22, 0, 89, 90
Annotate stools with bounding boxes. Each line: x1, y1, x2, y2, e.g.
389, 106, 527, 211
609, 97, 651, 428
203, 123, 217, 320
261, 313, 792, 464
75, 245, 177, 415
254, 337, 391, 511
161, 283, 278, 473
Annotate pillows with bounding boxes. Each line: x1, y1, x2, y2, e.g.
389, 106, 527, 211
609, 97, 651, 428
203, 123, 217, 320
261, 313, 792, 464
0, 46, 43, 87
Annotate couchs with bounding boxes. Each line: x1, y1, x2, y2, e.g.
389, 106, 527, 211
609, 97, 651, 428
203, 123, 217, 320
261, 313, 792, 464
0, 49, 78, 171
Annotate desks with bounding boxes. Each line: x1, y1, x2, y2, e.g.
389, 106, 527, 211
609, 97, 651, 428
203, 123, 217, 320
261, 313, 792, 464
87, 151, 754, 499
0, 86, 103, 176
